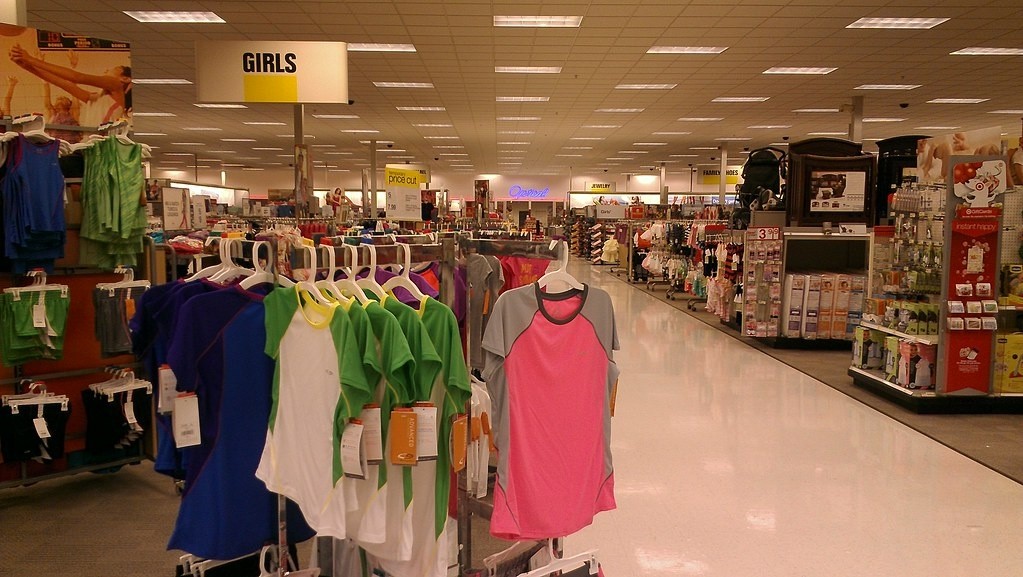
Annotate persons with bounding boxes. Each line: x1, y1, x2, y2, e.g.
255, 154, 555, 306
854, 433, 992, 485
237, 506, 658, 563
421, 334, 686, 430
422, 199, 434, 222
861, 329, 878, 368
296, 151, 307, 206
326, 192, 330, 205
909, 343, 922, 385
179, 188, 190, 229
917, 132, 999, 179
331, 187, 341, 217
599, 195, 620, 205
631, 196, 639, 205
672, 195, 705, 205
1005, 130, 1023, 188
145, 179, 160, 200
0, 44, 132, 143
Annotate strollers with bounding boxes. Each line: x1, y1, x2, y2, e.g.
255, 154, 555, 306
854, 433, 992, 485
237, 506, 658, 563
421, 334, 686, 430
726, 146, 786, 230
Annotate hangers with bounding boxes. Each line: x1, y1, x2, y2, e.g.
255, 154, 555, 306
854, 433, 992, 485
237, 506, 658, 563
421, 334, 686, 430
177, 218, 606, 576
3, 267, 154, 302
0, 115, 153, 159
2, 366, 153, 415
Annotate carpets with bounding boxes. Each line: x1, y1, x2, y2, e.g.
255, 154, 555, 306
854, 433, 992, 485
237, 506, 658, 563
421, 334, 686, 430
0, 460, 518, 577
608, 270, 1023, 486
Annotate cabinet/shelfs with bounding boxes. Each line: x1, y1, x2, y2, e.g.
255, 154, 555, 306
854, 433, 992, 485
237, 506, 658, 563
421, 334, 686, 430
847, 183, 1023, 415
569, 196, 869, 341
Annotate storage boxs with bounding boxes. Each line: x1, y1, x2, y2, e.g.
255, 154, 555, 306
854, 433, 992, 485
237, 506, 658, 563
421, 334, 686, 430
994, 330, 1023, 393
851, 326, 936, 390
1000, 263, 1023, 307
